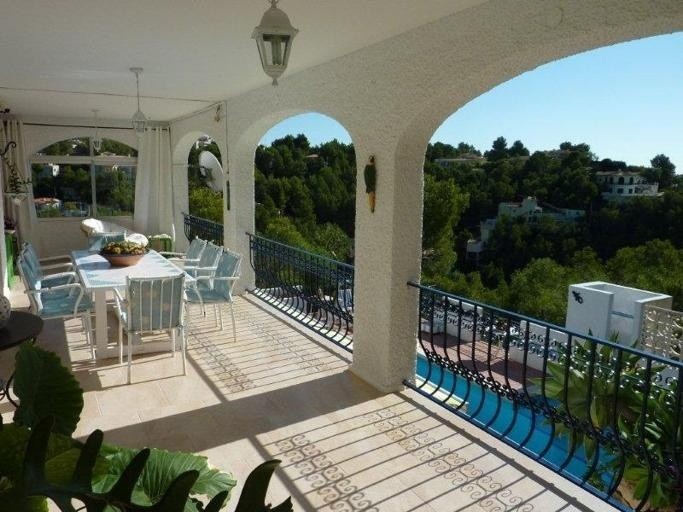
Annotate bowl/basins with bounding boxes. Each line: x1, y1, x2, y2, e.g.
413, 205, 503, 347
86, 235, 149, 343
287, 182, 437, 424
99, 247, 149, 266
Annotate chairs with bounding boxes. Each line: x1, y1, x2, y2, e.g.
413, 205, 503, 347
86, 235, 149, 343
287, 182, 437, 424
159, 237, 243, 344
17, 241, 94, 361
126, 272, 186, 385
89, 232, 126, 252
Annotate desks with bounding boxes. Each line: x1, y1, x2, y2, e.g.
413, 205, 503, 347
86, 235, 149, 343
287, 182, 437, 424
0, 306, 44, 414
68, 246, 197, 361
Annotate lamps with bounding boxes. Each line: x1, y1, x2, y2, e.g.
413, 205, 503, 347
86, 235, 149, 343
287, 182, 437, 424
90, 108, 103, 153
248, 0, 300, 88
128, 66, 148, 139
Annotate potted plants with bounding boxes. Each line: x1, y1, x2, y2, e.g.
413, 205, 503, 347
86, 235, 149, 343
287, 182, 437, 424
4, 215, 16, 235
98, 240, 149, 266
2, 151, 34, 206
